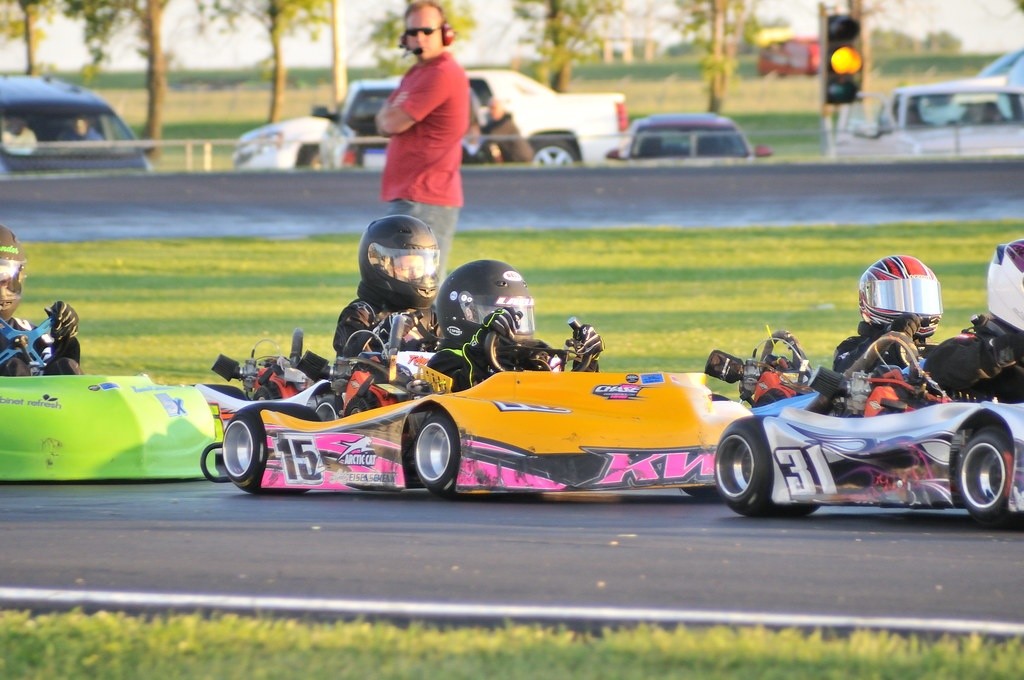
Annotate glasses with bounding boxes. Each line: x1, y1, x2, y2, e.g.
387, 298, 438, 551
406, 27, 441, 37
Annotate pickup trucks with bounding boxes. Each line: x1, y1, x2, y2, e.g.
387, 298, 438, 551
232, 69, 629, 166
312, 78, 504, 165
838, 81, 1024, 154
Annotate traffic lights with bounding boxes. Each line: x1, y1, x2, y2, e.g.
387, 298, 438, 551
823, 13, 861, 105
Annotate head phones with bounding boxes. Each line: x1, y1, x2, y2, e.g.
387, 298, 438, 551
399, 3, 455, 50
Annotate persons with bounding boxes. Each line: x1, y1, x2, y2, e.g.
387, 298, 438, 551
427, 260, 605, 392
376, 0, 470, 313
461, 95, 535, 164
0, 226, 83, 377
832, 256, 945, 372
332, 215, 442, 373
894, 101, 1008, 127
925, 239, 1024, 402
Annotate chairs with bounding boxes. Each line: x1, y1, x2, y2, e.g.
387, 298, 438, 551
640, 134, 663, 158
697, 133, 722, 154
906, 104, 922, 125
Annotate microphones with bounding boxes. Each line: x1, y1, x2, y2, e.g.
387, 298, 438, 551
400, 45, 424, 54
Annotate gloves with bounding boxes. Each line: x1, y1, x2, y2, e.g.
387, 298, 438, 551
565, 323, 605, 363
993, 329, 1023, 368
887, 313, 921, 340
44, 300, 80, 343
482, 305, 523, 339
372, 312, 416, 343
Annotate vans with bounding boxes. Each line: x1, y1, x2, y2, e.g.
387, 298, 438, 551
758, 37, 821, 77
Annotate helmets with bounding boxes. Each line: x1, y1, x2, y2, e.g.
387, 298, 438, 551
357, 214, 441, 311
858, 254, 944, 339
436, 259, 535, 348
986, 239, 1023, 331
0, 224, 28, 322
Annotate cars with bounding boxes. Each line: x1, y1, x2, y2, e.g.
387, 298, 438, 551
606, 113, 772, 159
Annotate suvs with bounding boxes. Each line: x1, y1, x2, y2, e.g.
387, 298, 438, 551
0, 73, 152, 174
